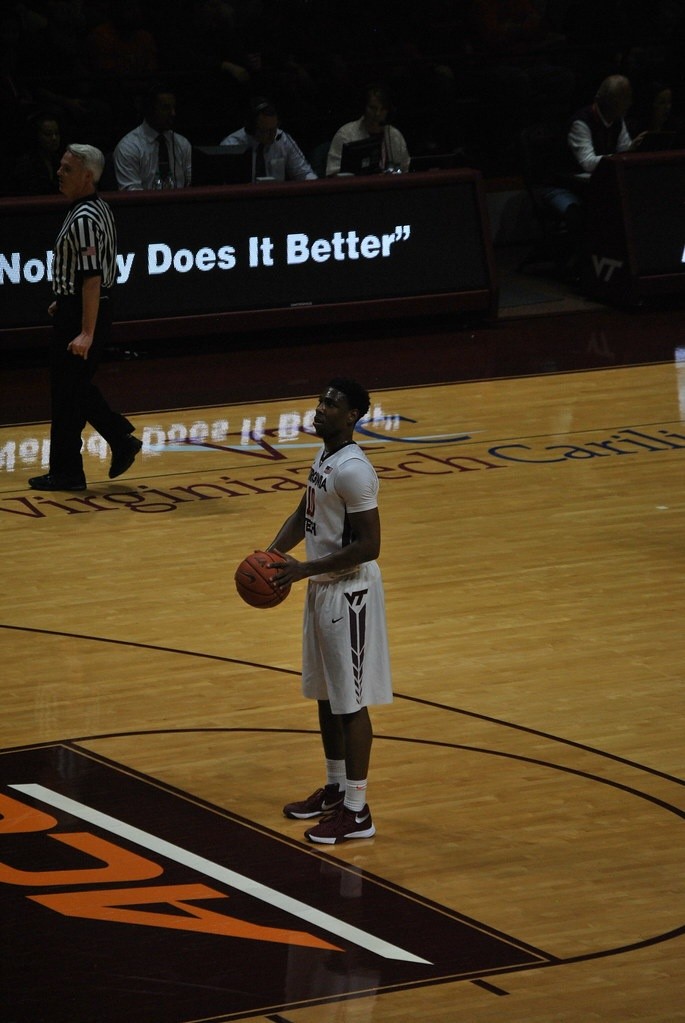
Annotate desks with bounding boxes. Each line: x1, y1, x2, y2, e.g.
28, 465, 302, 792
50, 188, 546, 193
573, 152, 685, 310
0, 168, 500, 352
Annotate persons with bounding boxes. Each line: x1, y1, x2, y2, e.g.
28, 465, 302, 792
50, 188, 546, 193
541, 74, 653, 314
219, 97, 320, 182
1, 1, 685, 136
112, 85, 193, 190
628, 80, 683, 149
251, 375, 391, 845
18, 110, 69, 194
27, 142, 144, 489
325, 82, 413, 175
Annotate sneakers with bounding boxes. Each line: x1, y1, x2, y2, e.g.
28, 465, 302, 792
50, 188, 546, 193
284, 783, 346, 820
305, 803, 376, 843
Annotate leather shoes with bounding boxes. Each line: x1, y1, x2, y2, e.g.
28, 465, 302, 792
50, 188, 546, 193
108, 435, 142, 478
29, 473, 87, 490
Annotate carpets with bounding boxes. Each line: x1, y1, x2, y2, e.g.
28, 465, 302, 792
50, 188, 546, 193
499, 277, 563, 311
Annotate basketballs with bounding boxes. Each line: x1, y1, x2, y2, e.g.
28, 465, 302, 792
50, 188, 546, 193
235, 552, 291, 608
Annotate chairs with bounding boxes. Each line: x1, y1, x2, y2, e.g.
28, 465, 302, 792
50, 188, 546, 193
519, 137, 576, 283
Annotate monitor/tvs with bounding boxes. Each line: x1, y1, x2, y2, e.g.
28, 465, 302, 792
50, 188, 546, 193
638, 130, 685, 153
340, 133, 383, 176
191, 144, 254, 186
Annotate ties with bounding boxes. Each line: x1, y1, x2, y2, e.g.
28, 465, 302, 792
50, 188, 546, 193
255, 144, 265, 177
155, 135, 172, 189
